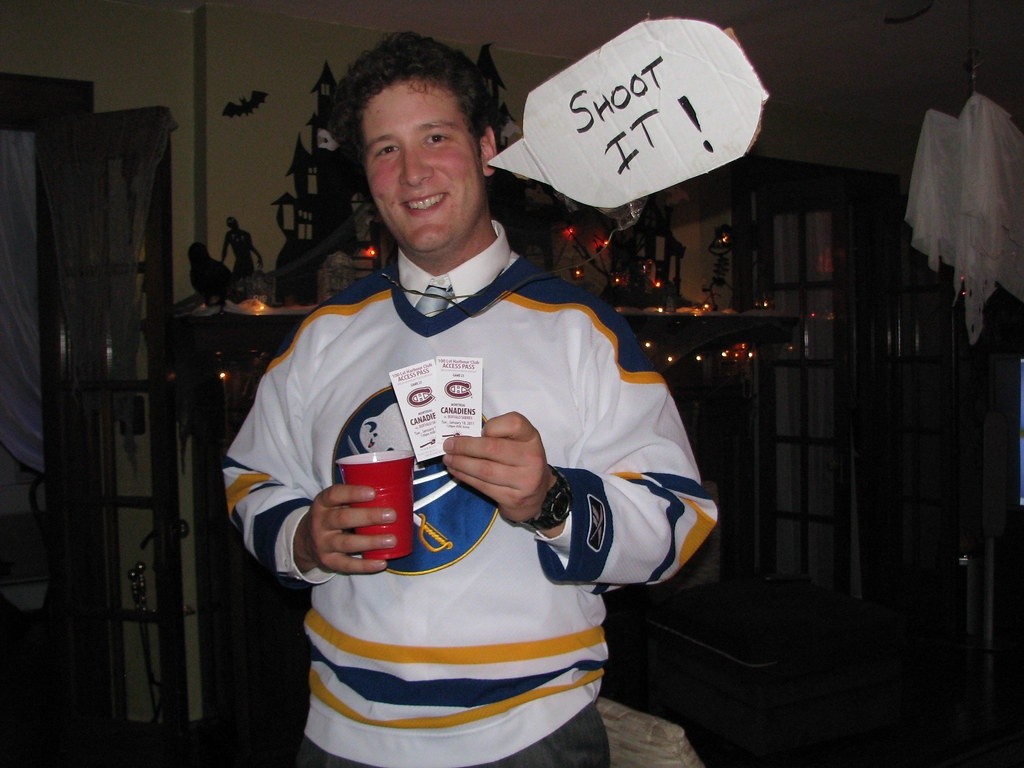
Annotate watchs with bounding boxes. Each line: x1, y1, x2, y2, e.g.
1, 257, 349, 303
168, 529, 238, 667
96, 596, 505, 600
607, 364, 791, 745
511, 464, 573, 530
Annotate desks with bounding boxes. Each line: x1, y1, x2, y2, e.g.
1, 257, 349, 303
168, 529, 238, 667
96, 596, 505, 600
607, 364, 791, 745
167, 313, 802, 711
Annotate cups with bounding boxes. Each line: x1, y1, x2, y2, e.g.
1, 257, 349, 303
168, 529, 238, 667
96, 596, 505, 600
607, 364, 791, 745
335, 451, 416, 559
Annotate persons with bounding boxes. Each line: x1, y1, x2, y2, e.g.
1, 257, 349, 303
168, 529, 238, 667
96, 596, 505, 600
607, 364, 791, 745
222, 34, 717, 768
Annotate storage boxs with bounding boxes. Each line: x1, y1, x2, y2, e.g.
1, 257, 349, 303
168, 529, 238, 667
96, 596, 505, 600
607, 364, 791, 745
644, 574, 900, 755
